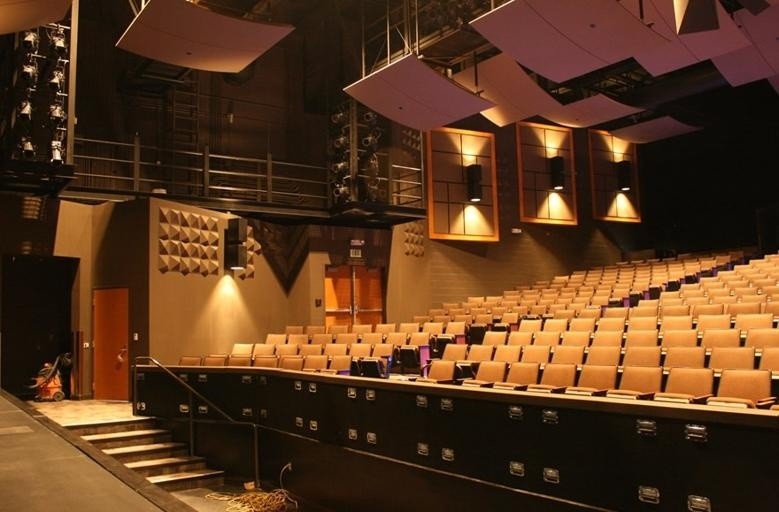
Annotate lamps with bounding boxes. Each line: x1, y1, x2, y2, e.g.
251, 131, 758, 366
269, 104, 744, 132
549, 156, 565, 190
227, 218, 248, 270
618, 160, 631, 191
467, 165, 483, 203
12, 22, 74, 171
330, 109, 378, 199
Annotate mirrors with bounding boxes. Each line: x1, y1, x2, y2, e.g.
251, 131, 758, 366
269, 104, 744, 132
425, 120, 643, 245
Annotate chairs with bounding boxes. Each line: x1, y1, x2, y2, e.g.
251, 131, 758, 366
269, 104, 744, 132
177, 246, 779, 409
